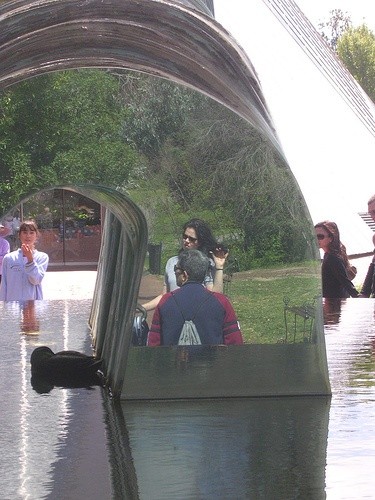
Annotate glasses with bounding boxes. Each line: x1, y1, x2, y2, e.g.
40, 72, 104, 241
317, 234, 331, 240
182, 234, 199, 244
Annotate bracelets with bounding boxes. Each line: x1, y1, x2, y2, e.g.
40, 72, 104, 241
215, 268, 223, 271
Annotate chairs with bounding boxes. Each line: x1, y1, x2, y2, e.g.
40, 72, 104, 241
277, 294, 320, 344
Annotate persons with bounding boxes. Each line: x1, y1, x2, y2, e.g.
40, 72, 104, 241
139, 218, 231, 314
0, 221, 49, 301
148, 249, 247, 345
357, 195, 375, 297
313, 220, 361, 299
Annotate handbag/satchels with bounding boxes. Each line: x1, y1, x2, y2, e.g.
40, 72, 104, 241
132, 304, 148, 347
